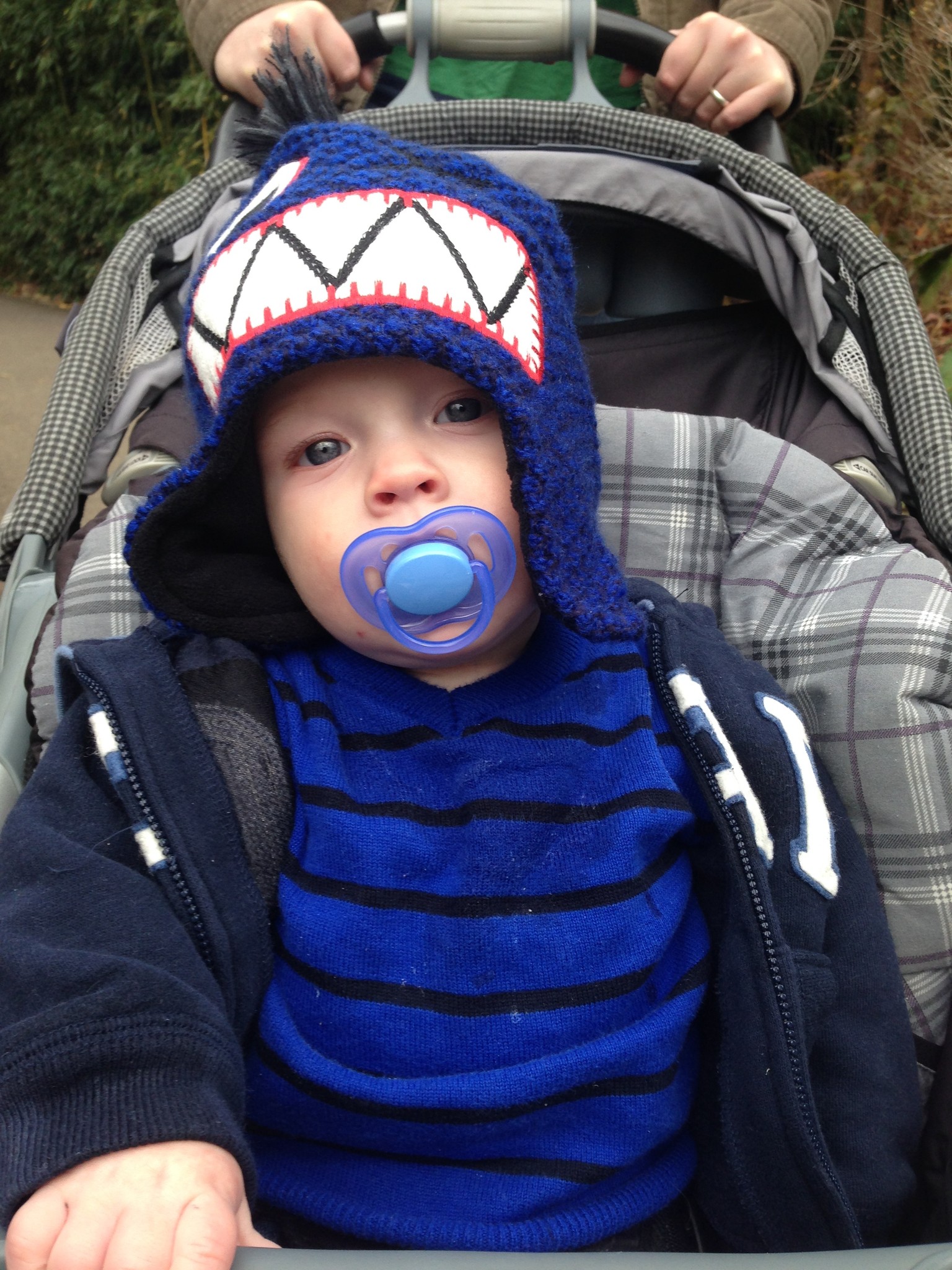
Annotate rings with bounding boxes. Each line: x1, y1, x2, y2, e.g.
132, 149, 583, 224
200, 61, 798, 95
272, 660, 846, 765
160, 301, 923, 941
709, 85, 730, 109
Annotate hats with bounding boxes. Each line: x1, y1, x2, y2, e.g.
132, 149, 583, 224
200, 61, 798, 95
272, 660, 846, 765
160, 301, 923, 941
118, 19, 648, 644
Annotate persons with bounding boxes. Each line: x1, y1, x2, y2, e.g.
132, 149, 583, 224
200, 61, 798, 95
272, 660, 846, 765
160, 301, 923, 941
0, 121, 945, 1270
168, 0, 847, 141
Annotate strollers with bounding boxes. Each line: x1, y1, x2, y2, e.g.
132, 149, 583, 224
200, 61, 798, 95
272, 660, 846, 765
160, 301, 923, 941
0, 0, 951, 1270
